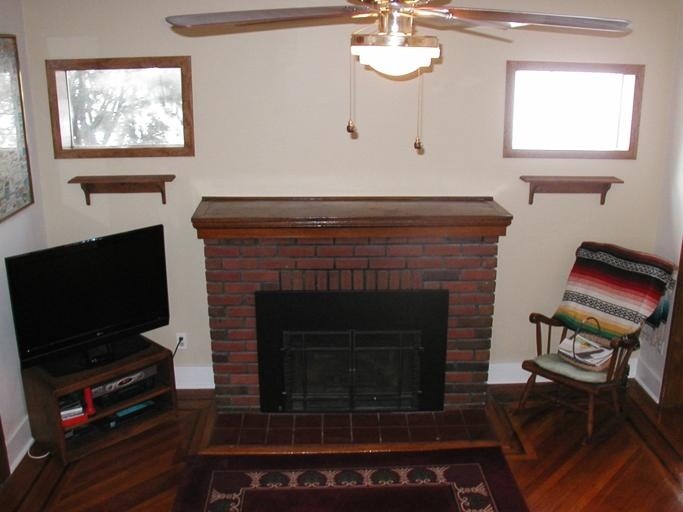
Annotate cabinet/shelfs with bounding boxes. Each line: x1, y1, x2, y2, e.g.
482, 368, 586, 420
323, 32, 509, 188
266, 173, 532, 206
20, 358, 176, 463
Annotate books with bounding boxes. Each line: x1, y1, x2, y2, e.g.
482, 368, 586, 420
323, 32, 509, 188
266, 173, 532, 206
58, 398, 84, 421
559, 334, 614, 367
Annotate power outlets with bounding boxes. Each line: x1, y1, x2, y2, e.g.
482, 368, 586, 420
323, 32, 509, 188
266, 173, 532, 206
174, 331, 187, 350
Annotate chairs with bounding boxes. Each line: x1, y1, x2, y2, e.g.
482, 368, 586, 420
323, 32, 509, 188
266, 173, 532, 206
510, 240, 676, 438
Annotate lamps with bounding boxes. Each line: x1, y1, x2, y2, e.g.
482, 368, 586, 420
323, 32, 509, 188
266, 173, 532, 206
350, 1, 440, 78
161, 2, 631, 43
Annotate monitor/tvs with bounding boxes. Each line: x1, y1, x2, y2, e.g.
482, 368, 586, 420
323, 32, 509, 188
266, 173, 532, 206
5, 224, 169, 378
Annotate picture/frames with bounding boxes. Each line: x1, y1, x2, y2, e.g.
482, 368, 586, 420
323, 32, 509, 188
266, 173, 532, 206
0, 36, 34, 227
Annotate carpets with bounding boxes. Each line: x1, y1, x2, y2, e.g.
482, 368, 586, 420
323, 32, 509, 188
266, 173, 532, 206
170, 446, 528, 511
